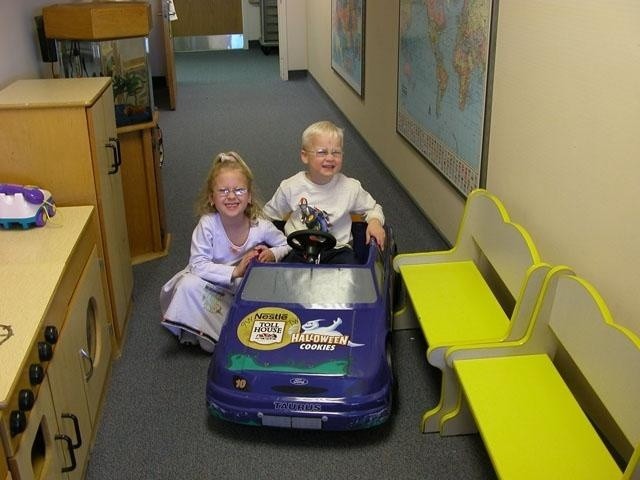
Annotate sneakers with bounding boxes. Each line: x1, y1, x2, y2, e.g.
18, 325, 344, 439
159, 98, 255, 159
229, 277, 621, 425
179, 329, 216, 353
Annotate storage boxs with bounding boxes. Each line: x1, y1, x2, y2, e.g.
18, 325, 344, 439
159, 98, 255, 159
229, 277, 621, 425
42, 0, 155, 128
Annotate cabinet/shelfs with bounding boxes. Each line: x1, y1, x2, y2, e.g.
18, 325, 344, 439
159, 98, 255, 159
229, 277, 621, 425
47, 206, 121, 436
116, 110, 170, 266
0, 77, 135, 355
0, 311, 95, 480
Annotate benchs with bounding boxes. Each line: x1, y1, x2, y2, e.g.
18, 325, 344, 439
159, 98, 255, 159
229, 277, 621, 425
440, 265, 640, 480
392, 189, 554, 433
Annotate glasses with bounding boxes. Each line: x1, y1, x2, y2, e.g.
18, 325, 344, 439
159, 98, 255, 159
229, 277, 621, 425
216, 186, 248, 197
304, 146, 343, 158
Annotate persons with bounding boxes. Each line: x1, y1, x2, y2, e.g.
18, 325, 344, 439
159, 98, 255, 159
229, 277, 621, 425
159, 150, 293, 354
258, 120, 386, 264
